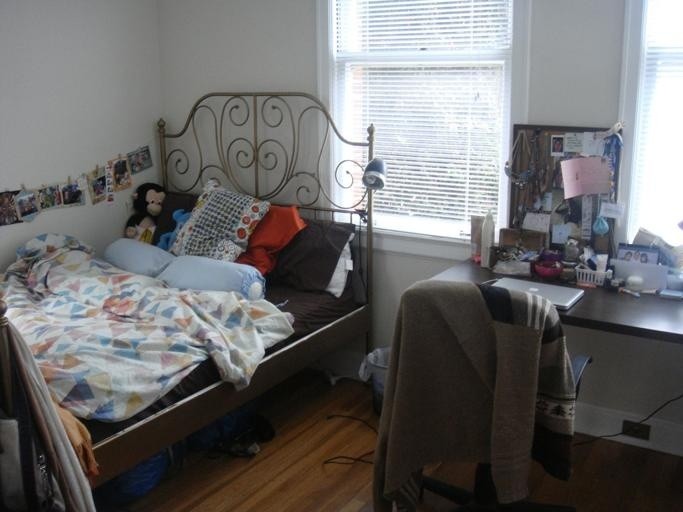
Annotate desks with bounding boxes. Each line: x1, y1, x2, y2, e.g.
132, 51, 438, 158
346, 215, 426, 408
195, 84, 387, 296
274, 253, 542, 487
426, 256, 682, 345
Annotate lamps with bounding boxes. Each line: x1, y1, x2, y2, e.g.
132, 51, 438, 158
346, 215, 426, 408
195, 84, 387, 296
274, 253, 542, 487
355, 158, 387, 223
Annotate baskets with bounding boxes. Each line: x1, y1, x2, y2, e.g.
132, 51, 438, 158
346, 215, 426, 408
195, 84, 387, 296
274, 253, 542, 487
575, 264, 608, 286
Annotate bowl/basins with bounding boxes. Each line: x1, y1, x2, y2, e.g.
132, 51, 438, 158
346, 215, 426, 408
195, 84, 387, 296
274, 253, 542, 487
535, 263, 563, 277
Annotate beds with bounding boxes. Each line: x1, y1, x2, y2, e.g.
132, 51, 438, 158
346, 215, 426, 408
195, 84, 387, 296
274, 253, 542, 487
2, 91, 375, 512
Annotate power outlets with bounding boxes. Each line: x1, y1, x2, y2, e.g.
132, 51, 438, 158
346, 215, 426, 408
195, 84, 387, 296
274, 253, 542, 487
622, 420, 652, 441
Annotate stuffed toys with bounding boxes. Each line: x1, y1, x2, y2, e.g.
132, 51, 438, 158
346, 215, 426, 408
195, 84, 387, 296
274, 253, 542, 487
126, 183, 176, 242
157, 209, 192, 251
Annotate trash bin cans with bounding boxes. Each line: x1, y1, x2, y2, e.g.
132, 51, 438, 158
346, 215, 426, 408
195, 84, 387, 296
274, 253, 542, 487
366, 346, 400, 414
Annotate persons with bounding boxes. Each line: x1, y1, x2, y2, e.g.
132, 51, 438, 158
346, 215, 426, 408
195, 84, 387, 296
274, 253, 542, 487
622, 250, 647, 263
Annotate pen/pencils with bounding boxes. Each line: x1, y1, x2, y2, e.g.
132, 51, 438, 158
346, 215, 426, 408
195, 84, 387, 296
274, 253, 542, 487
618, 287, 640, 298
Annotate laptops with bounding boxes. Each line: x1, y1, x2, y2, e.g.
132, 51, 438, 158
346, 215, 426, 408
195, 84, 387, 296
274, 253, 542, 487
491, 276, 585, 311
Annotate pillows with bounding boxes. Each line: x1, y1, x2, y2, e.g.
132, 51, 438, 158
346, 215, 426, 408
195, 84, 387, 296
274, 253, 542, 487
234, 205, 307, 277
265, 216, 357, 300
169, 178, 272, 263
104, 236, 266, 301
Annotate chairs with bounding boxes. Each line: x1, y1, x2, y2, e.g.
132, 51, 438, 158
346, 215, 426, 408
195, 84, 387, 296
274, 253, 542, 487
371, 280, 593, 512
